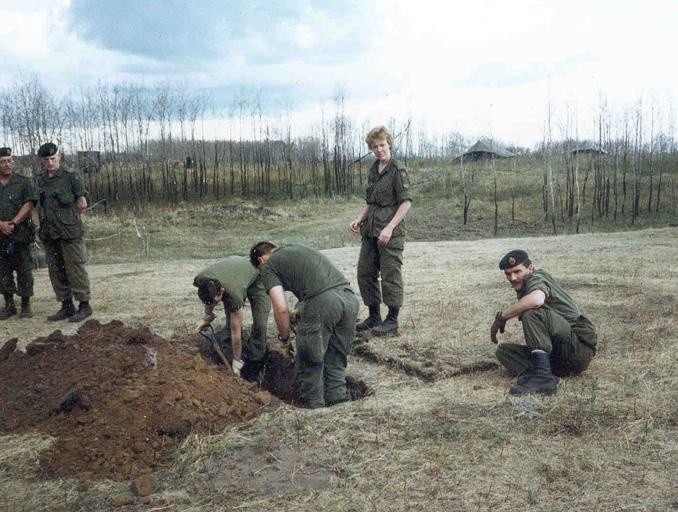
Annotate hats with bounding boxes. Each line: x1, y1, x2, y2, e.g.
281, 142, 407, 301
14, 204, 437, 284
500, 250, 527, 269
38, 143, 57, 157
0, 147, 13, 157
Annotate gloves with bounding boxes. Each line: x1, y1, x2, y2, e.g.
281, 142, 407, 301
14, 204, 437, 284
232, 359, 245, 377
489, 312, 506, 344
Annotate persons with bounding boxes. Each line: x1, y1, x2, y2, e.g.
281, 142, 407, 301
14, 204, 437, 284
348, 124, 413, 336
249, 240, 361, 407
490, 248, 598, 396
0, 147, 38, 319
191, 255, 270, 381
33, 141, 93, 322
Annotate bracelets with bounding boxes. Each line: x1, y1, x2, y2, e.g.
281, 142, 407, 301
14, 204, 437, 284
10, 221, 18, 229
277, 332, 290, 343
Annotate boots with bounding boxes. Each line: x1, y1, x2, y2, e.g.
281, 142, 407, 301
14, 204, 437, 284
509, 351, 558, 397
70, 300, 93, 323
373, 306, 400, 336
19, 295, 34, 319
0, 293, 18, 321
48, 298, 75, 322
356, 305, 380, 331
